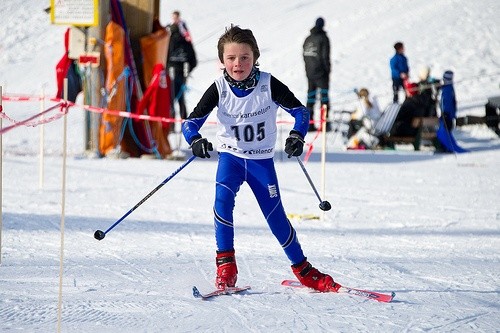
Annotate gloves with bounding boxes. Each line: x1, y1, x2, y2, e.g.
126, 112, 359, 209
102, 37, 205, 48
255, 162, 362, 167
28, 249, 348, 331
189, 134, 213, 159
284, 131, 305, 158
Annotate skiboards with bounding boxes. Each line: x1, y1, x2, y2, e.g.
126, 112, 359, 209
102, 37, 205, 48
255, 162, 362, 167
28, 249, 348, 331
281, 279, 396, 303
192, 285, 251, 300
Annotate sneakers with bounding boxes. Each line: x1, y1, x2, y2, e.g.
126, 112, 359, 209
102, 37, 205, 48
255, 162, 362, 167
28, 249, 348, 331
290, 256, 340, 291
215, 250, 238, 290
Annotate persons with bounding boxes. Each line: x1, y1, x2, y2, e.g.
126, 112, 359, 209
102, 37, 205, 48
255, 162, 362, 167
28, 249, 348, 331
390, 42, 409, 104
170, 11, 191, 44
436, 70, 457, 152
166, 23, 198, 133
344, 89, 381, 142
180, 24, 333, 295
302, 17, 332, 132
